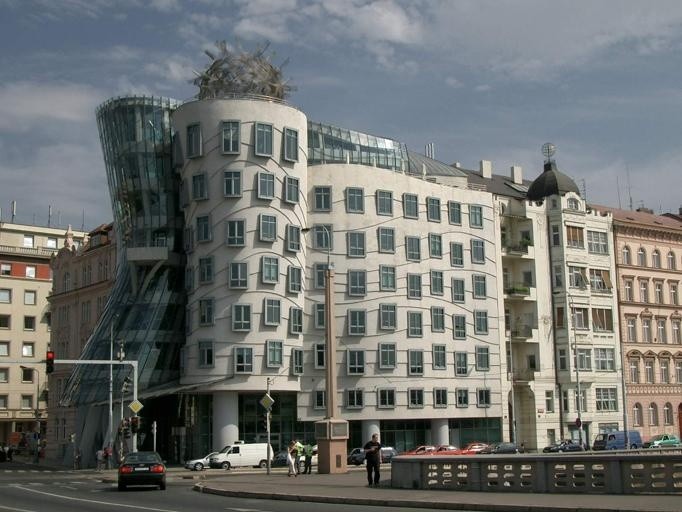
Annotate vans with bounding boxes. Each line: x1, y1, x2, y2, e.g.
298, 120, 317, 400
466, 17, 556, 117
210, 440, 275, 469
592, 430, 642, 452
297, 444, 320, 467
347, 446, 399, 464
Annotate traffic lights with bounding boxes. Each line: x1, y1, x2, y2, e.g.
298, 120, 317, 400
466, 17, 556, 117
46, 351, 55, 375
130, 416, 141, 434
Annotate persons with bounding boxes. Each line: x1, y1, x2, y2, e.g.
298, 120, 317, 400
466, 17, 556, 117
20, 432, 29, 449
292, 438, 304, 474
363, 432, 383, 487
300, 439, 314, 475
0, 439, 124, 472
285, 440, 299, 477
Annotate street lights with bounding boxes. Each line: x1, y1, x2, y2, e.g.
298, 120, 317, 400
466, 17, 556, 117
299, 223, 352, 475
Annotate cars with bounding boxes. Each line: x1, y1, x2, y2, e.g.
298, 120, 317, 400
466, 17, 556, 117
400, 438, 524, 455
542, 438, 588, 453
117, 451, 167, 491
184, 450, 220, 471
641, 433, 681, 450
